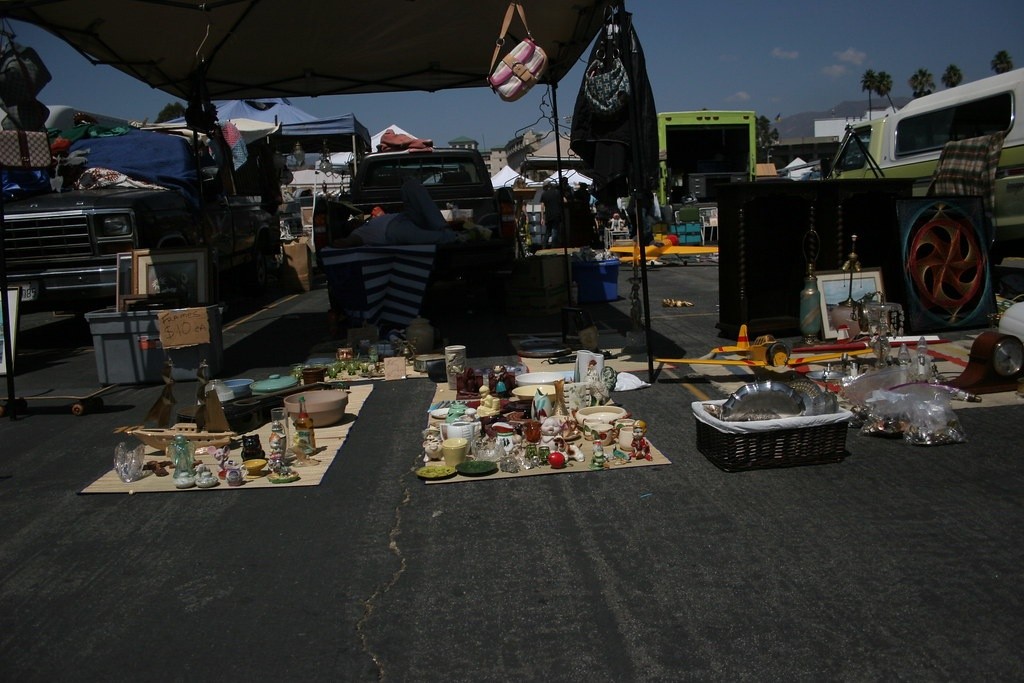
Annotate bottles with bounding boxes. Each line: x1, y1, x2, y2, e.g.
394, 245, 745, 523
294, 396, 316, 456
222, 378, 254, 394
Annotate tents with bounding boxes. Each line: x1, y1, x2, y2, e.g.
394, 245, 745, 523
311, 124, 419, 244
491, 165, 594, 212
172, 98, 372, 176
784, 158, 812, 179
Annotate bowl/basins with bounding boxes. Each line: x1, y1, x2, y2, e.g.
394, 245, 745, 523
241, 459, 267, 479
515, 371, 566, 386
283, 390, 349, 428
511, 384, 556, 402
575, 405, 627, 425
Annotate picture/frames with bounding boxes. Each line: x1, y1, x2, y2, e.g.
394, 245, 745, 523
132, 248, 207, 304
119, 293, 187, 311
0, 286, 23, 377
815, 266, 890, 339
116, 252, 132, 312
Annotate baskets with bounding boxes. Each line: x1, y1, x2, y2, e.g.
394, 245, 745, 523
695, 417, 849, 473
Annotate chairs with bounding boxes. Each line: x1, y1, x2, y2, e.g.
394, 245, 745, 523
439, 171, 471, 183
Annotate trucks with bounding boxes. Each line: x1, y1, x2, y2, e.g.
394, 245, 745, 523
820, 68, 1024, 269
0, 105, 131, 133
653, 108, 758, 214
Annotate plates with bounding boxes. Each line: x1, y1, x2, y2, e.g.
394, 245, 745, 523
415, 465, 457, 479
454, 460, 497, 476
431, 407, 449, 418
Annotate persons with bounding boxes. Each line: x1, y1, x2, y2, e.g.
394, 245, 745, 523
513, 199, 531, 246
173, 436, 193, 478
479, 386, 493, 407
269, 434, 285, 467
539, 177, 592, 250
331, 179, 492, 246
628, 420, 652, 461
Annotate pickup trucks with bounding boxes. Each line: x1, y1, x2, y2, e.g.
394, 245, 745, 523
313, 146, 518, 313
0, 134, 313, 304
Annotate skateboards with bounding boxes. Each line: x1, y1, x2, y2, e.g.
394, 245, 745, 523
0, 382, 119, 417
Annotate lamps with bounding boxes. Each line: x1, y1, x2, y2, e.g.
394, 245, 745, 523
318, 137, 335, 172
832, 234, 862, 341
293, 140, 305, 162
800, 206, 820, 345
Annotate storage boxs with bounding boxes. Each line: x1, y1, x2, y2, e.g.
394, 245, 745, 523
574, 259, 622, 302
283, 236, 316, 294
671, 221, 703, 245
84, 301, 228, 385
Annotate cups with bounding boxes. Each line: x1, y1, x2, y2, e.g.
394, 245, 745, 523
442, 437, 468, 467
225, 465, 247, 487
619, 427, 633, 451
615, 419, 635, 428
270, 407, 290, 454
338, 347, 353, 359
